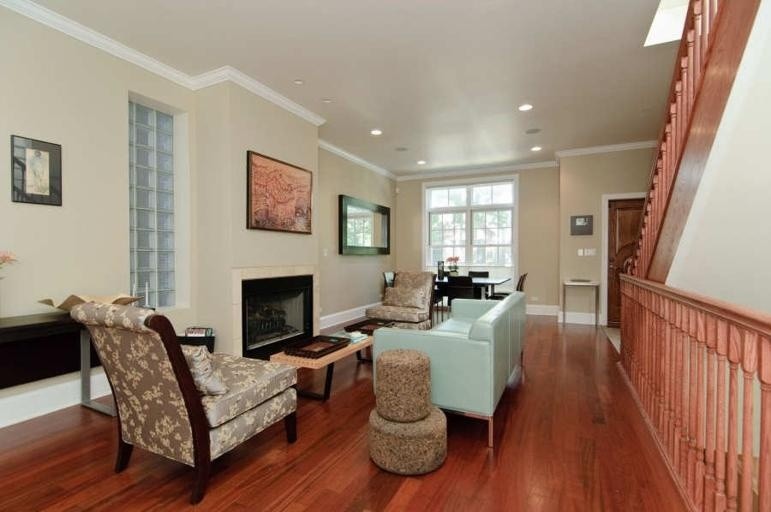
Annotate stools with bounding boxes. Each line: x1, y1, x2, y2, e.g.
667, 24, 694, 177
368, 347, 449, 475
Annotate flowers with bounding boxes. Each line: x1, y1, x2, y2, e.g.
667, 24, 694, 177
447, 256, 459, 272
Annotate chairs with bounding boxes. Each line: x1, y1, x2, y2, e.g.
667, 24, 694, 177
69, 302, 298, 506
366, 271, 436, 361
430, 270, 527, 323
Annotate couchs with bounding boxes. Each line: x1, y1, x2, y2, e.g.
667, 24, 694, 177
371, 289, 527, 450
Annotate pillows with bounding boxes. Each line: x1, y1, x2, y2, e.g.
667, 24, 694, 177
179, 344, 231, 397
384, 286, 427, 308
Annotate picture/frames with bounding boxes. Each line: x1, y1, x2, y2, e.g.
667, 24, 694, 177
11, 134, 62, 208
338, 194, 391, 256
570, 216, 594, 236
246, 150, 314, 235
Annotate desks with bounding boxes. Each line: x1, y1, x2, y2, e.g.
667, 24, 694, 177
561, 277, 600, 331
0, 310, 118, 391
